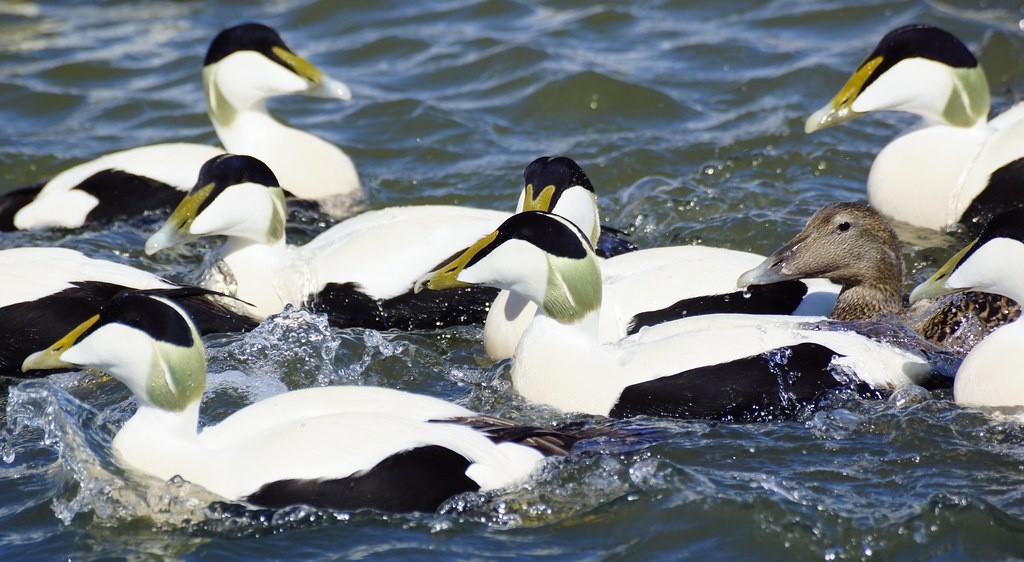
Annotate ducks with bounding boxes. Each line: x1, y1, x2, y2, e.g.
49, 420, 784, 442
0, 22, 1024, 518
